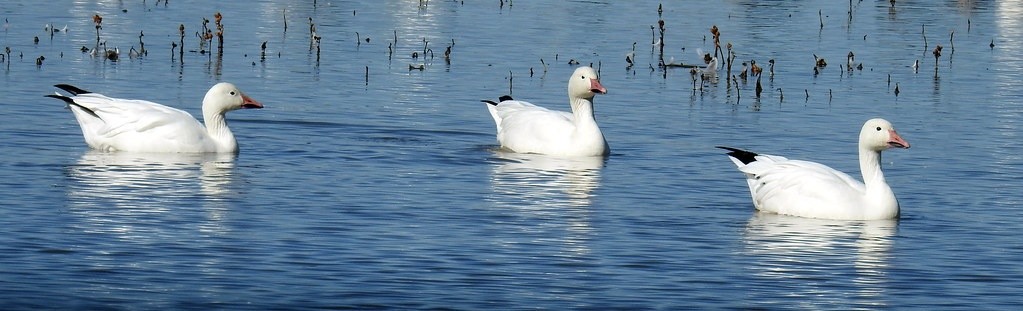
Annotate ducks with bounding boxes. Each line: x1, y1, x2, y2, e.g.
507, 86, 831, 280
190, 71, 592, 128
44, 81, 263, 155
478, 65, 611, 159
713, 117, 912, 221
